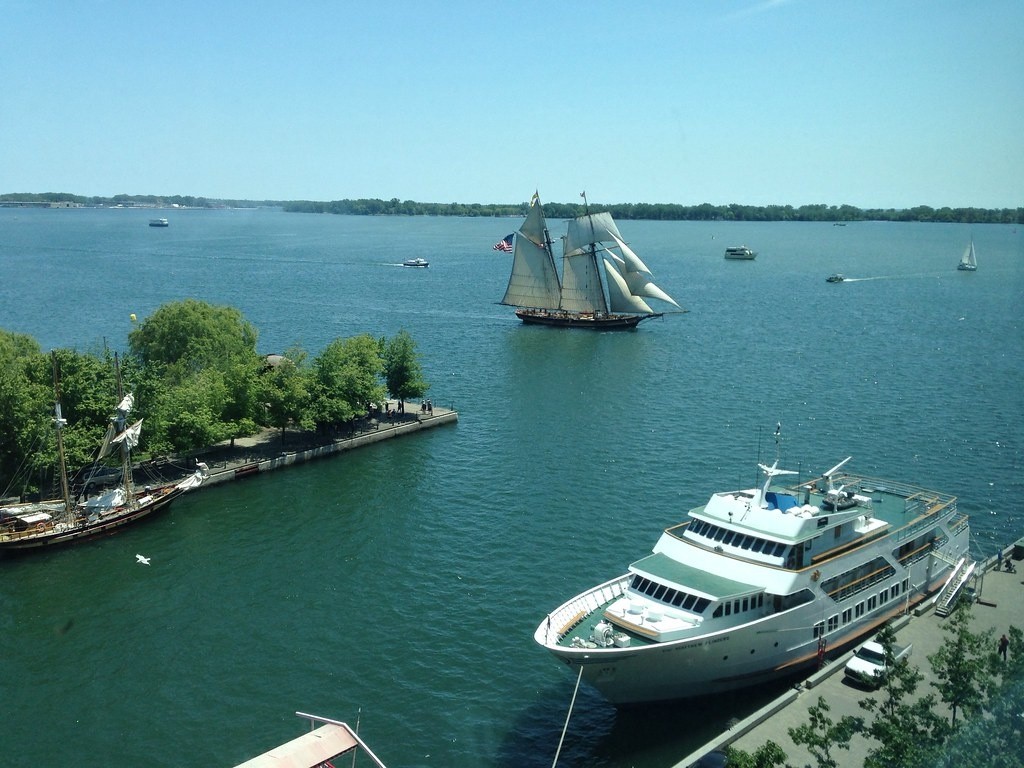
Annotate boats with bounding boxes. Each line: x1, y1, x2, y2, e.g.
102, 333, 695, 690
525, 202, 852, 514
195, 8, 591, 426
724, 245, 759, 260
825, 273, 844, 283
149, 217, 169, 228
402, 257, 430, 267
533, 421, 971, 707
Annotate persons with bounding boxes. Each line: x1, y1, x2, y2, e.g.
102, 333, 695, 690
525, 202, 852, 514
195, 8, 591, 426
385, 402, 388, 412
387, 409, 396, 418
421, 399, 433, 416
997, 634, 1009, 662
397, 401, 402, 413
993, 549, 1004, 572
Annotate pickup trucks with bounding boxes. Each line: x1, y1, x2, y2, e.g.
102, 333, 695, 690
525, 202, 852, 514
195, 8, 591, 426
842, 638, 914, 691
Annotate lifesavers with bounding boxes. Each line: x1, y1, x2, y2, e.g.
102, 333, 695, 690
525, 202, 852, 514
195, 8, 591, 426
36, 522, 46, 533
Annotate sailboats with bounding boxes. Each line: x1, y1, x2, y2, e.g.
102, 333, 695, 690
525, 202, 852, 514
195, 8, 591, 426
493, 189, 692, 329
957, 233, 979, 271
0, 336, 210, 551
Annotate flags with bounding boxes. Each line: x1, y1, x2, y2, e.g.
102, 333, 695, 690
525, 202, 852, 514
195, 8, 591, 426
492, 233, 514, 254
